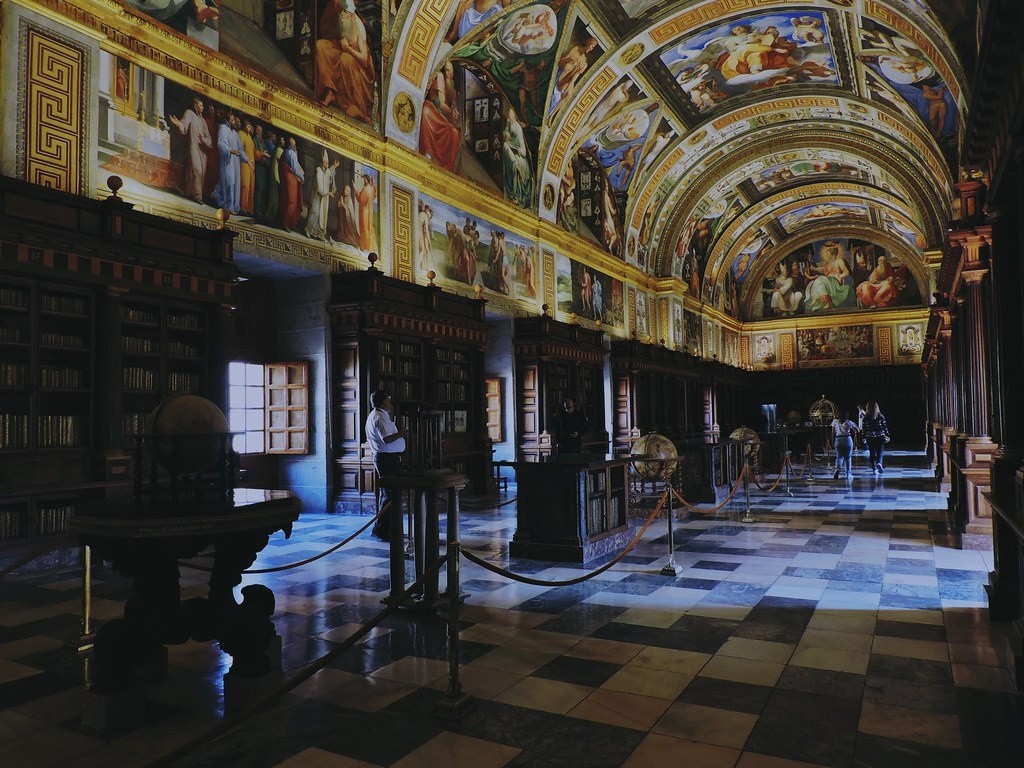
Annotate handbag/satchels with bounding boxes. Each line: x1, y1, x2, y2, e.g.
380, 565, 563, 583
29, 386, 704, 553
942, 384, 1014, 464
882, 435, 890, 443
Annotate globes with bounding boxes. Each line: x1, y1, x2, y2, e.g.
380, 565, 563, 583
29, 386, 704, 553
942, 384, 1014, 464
809, 394, 840, 429
630, 433, 679, 482
144, 392, 231, 477
729, 427, 761, 460
786, 410, 802, 424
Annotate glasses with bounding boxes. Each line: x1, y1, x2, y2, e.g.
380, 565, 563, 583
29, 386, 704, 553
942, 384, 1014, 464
383, 396, 392, 400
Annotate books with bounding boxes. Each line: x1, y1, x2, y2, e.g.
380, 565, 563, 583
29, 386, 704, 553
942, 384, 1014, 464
546, 365, 595, 407
377, 338, 472, 403
0, 286, 203, 541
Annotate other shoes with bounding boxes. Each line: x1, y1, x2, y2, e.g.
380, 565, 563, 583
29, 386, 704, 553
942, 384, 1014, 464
834, 470, 839, 480
847, 475, 853, 479
877, 464, 883, 474
872, 469, 877, 475
371, 531, 390, 541
859, 450, 870, 454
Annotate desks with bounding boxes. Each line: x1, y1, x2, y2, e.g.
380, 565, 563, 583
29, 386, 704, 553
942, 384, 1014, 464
64, 488, 302, 735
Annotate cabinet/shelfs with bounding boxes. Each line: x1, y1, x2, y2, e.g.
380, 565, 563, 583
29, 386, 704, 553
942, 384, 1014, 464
339, 327, 500, 515
0, 262, 231, 559
517, 359, 748, 488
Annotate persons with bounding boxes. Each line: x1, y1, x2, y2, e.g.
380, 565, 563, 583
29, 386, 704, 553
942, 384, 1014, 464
365, 390, 407, 541
554, 397, 589, 454
862, 400, 891, 474
830, 410, 860, 480
858, 404, 869, 453
